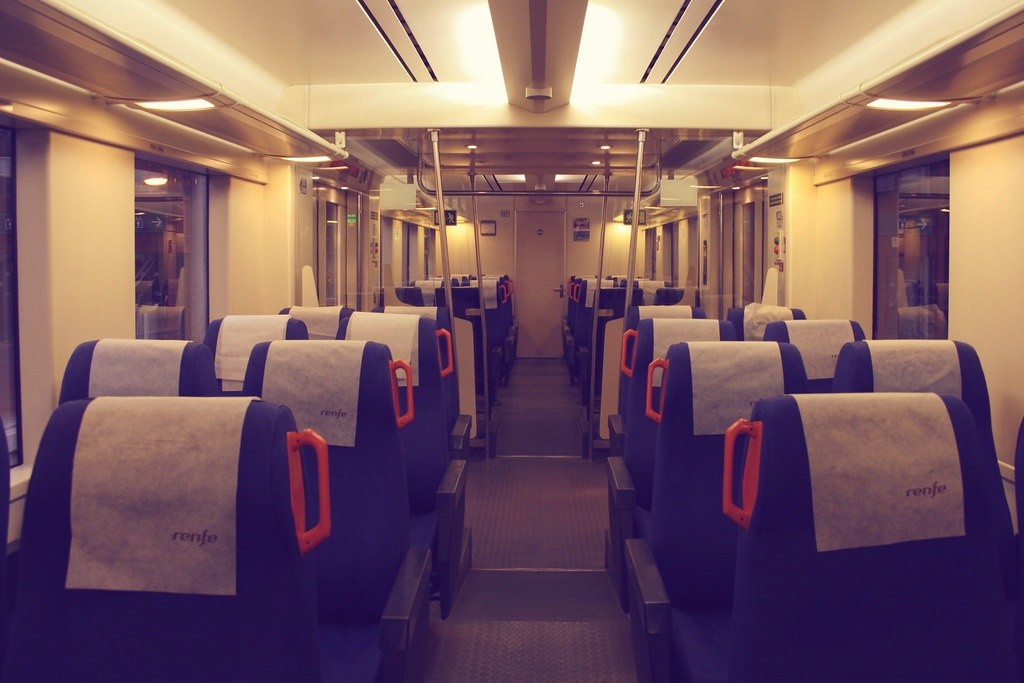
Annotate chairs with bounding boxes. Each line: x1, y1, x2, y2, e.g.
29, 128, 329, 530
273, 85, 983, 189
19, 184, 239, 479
0, 273, 517, 683
135, 267, 186, 341
561, 275, 1024, 683
897, 268, 948, 340
